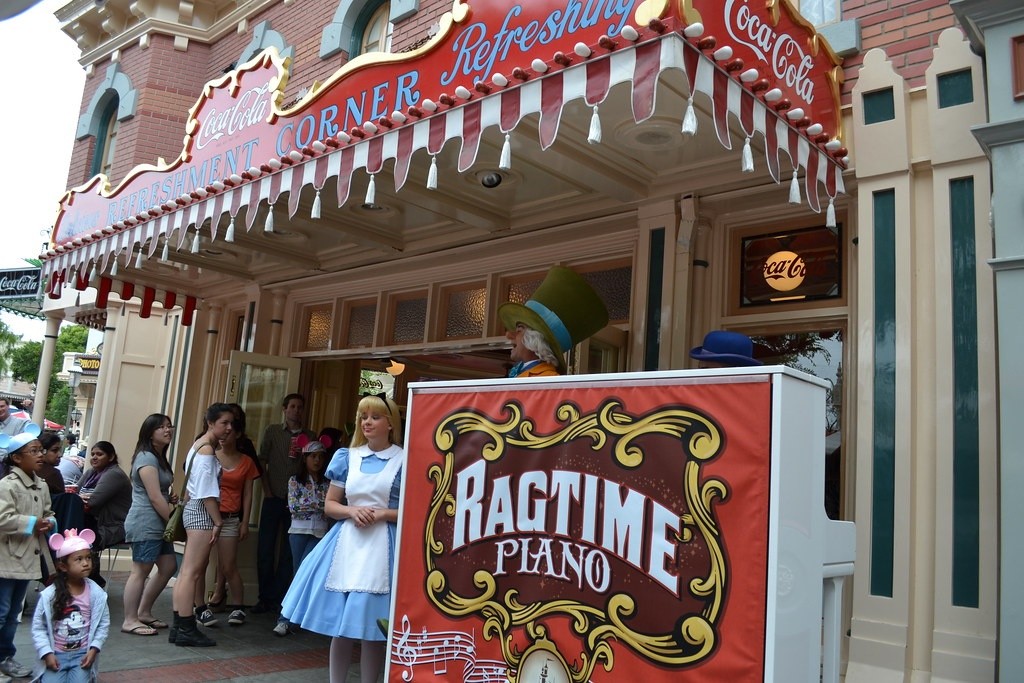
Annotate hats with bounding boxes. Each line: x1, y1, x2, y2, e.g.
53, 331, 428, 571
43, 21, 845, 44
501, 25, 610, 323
49, 528, 95, 558
689, 330, 761, 366
0, 423, 40, 454
296, 434, 331, 452
498, 266, 610, 375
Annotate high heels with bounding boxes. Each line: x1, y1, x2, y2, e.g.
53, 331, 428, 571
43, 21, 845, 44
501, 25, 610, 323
207, 590, 227, 613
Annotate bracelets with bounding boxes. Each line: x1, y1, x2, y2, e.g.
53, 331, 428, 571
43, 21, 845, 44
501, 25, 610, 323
215, 525, 222, 527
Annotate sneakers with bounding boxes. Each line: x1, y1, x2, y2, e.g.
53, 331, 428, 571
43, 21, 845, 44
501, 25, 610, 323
273, 623, 288, 636
0, 656, 33, 683
228, 609, 247, 624
196, 608, 218, 627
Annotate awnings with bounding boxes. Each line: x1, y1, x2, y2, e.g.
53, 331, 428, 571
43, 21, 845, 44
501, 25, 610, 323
0, 266, 46, 322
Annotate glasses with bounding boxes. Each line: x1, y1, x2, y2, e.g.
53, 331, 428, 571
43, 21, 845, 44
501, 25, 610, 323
158, 426, 176, 431
22, 449, 47, 455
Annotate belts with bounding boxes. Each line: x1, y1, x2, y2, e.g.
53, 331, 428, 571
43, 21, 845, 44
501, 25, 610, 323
227, 513, 238, 518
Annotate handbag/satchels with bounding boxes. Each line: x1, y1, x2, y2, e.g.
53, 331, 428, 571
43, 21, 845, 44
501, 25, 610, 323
162, 504, 187, 542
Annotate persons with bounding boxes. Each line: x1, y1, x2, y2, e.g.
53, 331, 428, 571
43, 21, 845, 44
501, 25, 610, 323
689, 331, 763, 369
0, 423, 58, 678
31, 529, 110, 683
281, 392, 404, 683
272, 434, 332, 637
0, 397, 31, 480
122, 413, 179, 636
55, 449, 87, 485
182, 404, 260, 626
168, 402, 234, 647
34, 432, 108, 588
73, 441, 133, 575
255, 394, 317, 612
496, 266, 609, 378
62, 434, 78, 456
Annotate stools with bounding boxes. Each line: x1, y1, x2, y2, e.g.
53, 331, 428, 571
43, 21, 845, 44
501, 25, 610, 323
104, 544, 132, 591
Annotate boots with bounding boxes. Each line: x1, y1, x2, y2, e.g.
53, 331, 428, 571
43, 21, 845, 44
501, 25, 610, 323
169, 611, 216, 647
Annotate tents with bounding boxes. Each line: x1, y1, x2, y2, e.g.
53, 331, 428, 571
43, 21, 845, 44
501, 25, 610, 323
7, 405, 63, 431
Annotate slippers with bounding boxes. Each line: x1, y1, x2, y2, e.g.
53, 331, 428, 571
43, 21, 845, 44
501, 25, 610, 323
121, 625, 158, 635
145, 619, 168, 629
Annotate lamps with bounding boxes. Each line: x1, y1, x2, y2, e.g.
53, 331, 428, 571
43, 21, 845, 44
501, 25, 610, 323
70, 406, 84, 426
